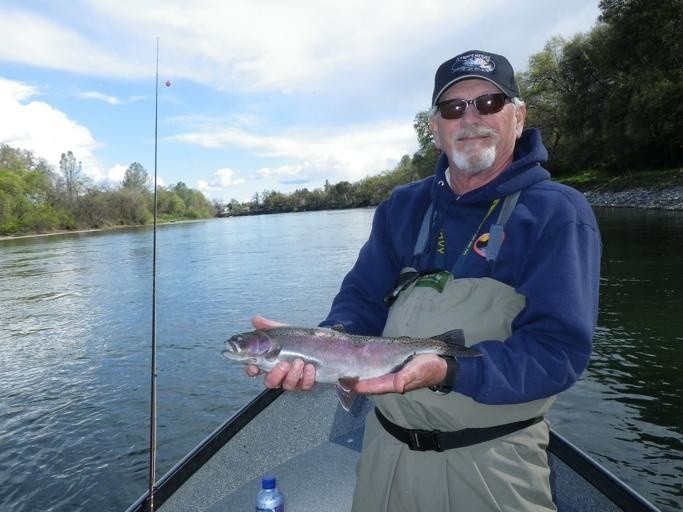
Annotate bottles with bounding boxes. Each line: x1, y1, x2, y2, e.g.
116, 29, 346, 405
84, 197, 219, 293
254, 476, 285, 512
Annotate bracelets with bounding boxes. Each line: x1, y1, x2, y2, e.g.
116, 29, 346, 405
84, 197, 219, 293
428, 355, 457, 395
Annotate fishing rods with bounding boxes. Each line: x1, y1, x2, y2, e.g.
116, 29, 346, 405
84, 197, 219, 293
151, 33, 171, 511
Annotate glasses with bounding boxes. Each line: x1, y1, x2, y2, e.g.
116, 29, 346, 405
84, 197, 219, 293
434, 92, 512, 120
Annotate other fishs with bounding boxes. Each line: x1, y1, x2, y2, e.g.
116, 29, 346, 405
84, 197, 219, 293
221, 324, 487, 411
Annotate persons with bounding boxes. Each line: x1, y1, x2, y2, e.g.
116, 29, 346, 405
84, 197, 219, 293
245, 50, 604, 512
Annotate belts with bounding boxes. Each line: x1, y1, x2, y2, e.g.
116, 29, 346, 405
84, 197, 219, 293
372, 401, 547, 456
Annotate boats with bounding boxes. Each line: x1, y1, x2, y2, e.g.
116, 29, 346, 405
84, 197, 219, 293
121, 388, 666, 512
218, 201, 272, 217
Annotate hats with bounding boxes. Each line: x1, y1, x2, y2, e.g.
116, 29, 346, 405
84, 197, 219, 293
429, 48, 520, 109
